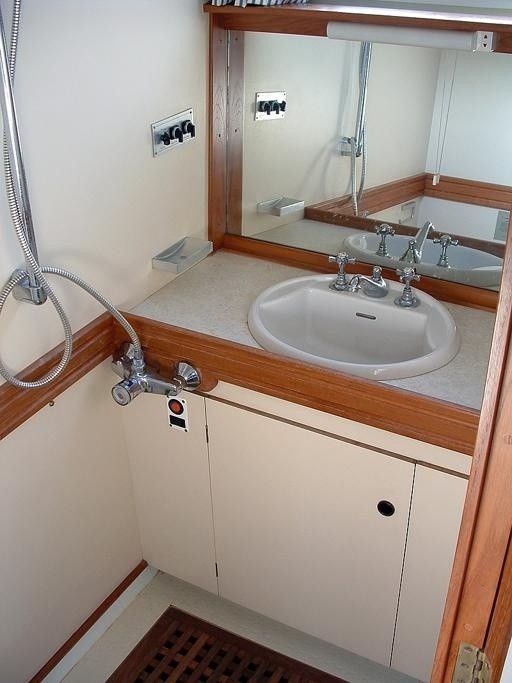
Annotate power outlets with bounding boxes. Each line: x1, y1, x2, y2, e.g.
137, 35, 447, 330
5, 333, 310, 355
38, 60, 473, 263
472, 31, 497, 54
476, 31, 497, 53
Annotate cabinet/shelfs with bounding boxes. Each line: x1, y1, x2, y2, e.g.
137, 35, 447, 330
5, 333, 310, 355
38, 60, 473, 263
111, 378, 474, 683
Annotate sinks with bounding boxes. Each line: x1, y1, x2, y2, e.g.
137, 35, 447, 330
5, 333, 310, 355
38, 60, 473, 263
343, 232, 504, 287
247, 273, 460, 380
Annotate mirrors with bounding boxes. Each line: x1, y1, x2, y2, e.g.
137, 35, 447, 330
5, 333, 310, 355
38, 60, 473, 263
203, 2, 512, 314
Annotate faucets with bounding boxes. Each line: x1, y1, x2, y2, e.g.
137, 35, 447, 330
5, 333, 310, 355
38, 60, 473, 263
346, 266, 389, 297
400, 220, 435, 263
